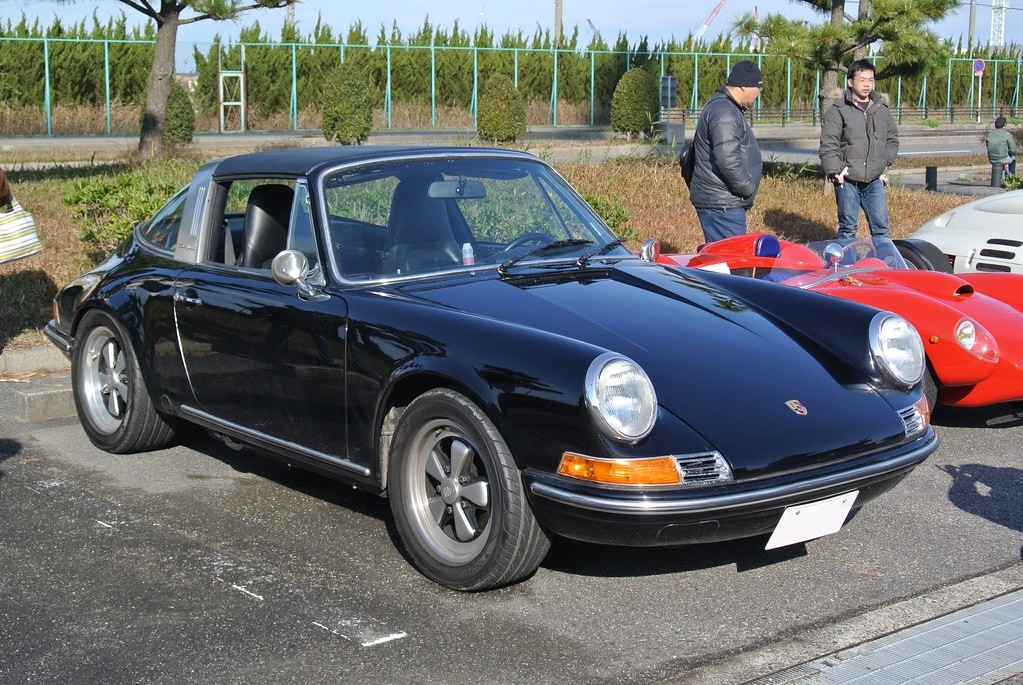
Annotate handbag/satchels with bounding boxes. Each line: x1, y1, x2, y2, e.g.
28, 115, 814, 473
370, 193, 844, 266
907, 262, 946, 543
0, 192, 43, 264
680, 143, 695, 190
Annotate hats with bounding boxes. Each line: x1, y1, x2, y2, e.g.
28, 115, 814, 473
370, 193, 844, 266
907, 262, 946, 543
728, 60, 763, 88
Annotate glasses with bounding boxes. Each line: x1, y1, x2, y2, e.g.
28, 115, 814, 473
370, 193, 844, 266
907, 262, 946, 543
854, 77, 875, 84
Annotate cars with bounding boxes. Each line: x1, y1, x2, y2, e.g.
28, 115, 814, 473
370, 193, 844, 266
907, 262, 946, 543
910, 188, 1023, 275
41, 146, 941, 593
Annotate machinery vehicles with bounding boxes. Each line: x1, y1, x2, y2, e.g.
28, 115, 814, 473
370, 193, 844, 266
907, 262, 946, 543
682, 0, 726, 50
754, 4, 761, 53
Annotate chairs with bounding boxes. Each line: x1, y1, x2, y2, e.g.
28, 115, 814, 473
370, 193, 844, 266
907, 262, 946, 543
383, 170, 483, 279
244, 184, 317, 274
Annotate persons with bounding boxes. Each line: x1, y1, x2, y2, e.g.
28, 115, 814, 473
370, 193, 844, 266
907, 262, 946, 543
985, 117, 1017, 174
687, 60, 763, 243
818, 57, 901, 237
0, 168, 12, 208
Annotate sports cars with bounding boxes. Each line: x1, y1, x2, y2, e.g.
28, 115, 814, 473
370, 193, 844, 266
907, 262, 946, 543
624, 232, 1023, 409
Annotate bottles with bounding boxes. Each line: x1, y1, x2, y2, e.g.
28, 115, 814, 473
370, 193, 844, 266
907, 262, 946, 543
462, 243, 474, 266
